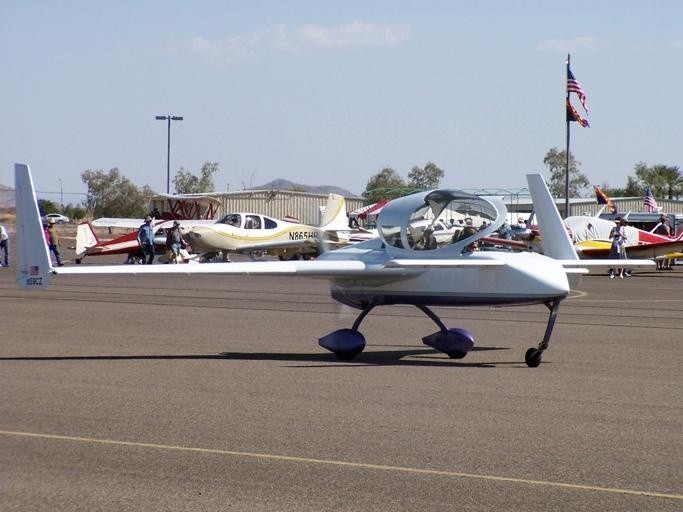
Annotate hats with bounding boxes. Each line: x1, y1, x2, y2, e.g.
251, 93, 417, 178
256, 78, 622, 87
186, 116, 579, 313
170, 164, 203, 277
49, 218, 56, 224
174, 221, 180, 225
615, 219, 621, 224
144, 216, 152, 222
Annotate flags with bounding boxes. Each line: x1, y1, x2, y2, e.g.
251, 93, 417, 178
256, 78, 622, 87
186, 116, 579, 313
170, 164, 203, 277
566, 69, 591, 128
595, 187, 617, 214
644, 188, 657, 209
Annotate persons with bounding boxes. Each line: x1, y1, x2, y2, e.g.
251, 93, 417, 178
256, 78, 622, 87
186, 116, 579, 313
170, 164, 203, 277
609, 217, 631, 279
43, 217, 64, 266
448, 217, 531, 251
0, 225, 9, 267
656, 215, 674, 270
167, 220, 188, 264
424, 229, 437, 249
137, 215, 155, 264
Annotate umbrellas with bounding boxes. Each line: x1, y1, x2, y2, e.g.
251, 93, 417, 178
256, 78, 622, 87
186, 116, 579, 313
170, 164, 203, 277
349, 199, 390, 220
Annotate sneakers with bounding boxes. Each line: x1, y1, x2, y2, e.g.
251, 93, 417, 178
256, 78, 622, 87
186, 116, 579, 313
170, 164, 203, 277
659, 267, 673, 270
609, 273, 631, 279
58, 263, 64, 266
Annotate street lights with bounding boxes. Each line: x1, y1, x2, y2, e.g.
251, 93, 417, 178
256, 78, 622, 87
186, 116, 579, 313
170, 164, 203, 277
156, 115, 183, 194
